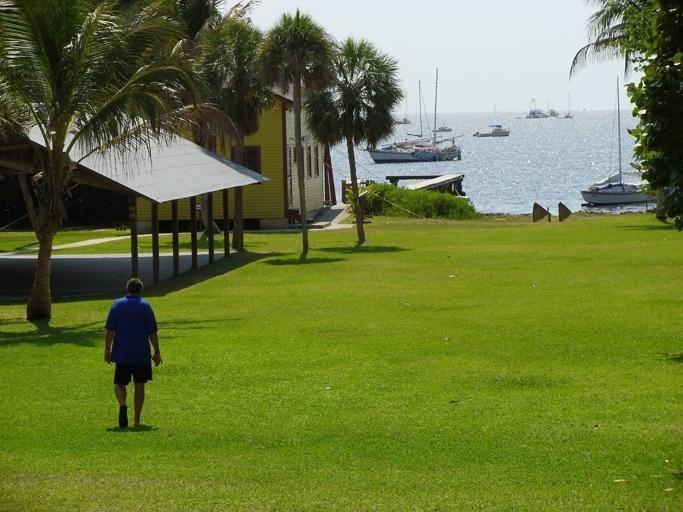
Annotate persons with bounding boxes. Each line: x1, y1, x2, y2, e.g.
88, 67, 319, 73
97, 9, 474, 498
103, 277, 164, 428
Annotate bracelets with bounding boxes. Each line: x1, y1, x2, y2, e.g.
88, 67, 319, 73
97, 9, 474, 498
155, 351, 159, 353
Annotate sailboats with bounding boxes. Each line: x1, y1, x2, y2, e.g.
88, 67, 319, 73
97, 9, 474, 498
366, 62, 462, 163
394, 90, 412, 125
579, 75, 676, 205
526, 92, 574, 120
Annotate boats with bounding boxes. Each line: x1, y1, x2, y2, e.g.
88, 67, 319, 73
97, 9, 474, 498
474, 124, 510, 137
432, 124, 452, 133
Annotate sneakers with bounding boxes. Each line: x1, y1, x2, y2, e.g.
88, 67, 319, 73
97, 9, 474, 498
119, 405, 128, 428
